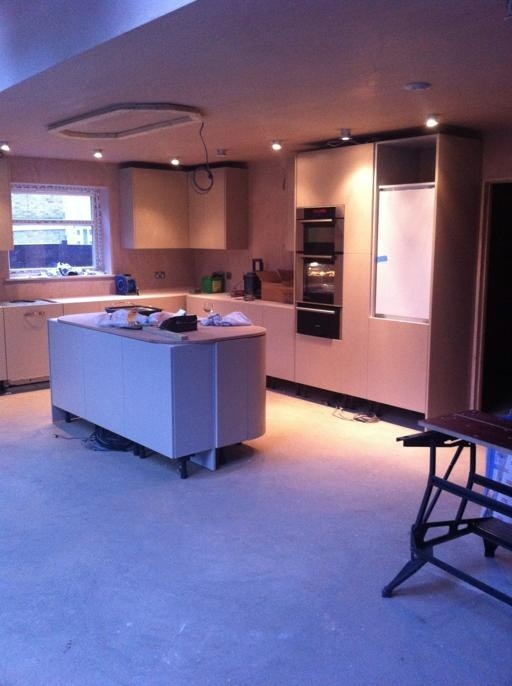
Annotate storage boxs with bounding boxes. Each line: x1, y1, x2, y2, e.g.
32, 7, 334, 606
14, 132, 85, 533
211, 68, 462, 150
255, 271, 294, 304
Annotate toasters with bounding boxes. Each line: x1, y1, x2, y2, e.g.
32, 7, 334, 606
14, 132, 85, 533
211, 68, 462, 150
115, 273, 138, 296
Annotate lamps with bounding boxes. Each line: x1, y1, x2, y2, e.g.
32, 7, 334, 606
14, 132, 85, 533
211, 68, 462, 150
169, 157, 180, 166
340, 128, 352, 141
215, 149, 226, 157
0, 141, 9, 152
272, 140, 282, 151
426, 113, 439, 128
93, 149, 103, 159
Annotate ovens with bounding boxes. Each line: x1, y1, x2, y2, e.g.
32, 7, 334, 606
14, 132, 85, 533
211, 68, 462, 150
294, 205, 344, 340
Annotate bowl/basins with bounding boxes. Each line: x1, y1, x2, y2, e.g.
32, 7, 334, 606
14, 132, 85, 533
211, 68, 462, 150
104, 305, 161, 316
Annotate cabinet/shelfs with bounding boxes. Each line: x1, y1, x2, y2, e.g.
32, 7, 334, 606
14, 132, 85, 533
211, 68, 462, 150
119, 167, 188, 251
188, 166, 249, 251
366, 316, 429, 415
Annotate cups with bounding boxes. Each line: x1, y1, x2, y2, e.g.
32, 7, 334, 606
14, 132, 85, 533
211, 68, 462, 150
203, 302, 213, 315
126, 311, 137, 327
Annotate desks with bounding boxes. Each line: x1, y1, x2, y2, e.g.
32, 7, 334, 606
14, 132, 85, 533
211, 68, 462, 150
382, 409, 512, 607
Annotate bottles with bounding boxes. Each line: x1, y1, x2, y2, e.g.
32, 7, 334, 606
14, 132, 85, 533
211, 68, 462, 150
243, 272, 260, 299
